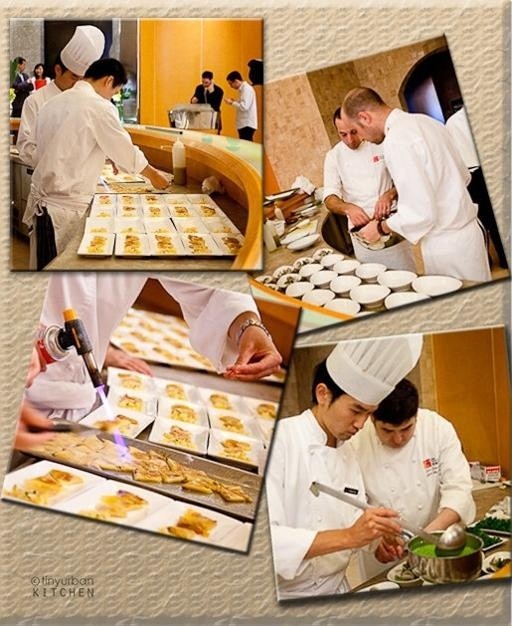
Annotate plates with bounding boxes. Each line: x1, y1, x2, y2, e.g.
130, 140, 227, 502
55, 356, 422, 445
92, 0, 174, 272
466, 519, 511, 536
100, 162, 174, 194
109, 306, 280, 381
280, 233, 309, 247
1, 457, 254, 552
481, 550, 512, 574
385, 560, 425, 586
289, 197, 319, 218
479, 534, 509, 553
476, 573, 493, 580
288, 234, 320, 252
355, 580, 400, 592
78, 365, 278, 476
82, 192, 249, 256
265, 191, 293, 203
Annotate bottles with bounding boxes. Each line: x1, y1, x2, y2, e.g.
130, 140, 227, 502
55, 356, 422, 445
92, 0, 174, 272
171, 138, 188, 186
263, 223, 278, 253
264, 218, 280, 245
272, 202, 284, 223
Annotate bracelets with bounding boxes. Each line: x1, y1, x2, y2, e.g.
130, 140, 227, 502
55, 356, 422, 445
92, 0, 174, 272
377, 219, 390, 235
235, 319, 273, 350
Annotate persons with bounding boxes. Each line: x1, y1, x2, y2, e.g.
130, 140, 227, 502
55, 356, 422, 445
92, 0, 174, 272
445, 104, 508, 270
16, 59, 84, 168
12, 57, 33, 117
29, 63, 50, 93
323, 107, 419, 275
191, 71, 223, 130
14, 350, 55, 449
349, 379, 476, 582
265, 361, 405, 600
341, 87, 493, 281
27, 272, 282, 423
224, 71, 258, 141
22, 58, 172, 269
103, 345, 155, 378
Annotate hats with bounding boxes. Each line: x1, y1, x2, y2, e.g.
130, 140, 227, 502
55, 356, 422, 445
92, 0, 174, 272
61, 25, 106, 77
326, 335, 424, 406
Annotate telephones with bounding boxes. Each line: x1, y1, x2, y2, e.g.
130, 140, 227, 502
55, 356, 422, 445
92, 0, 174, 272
203, 79, 215, 92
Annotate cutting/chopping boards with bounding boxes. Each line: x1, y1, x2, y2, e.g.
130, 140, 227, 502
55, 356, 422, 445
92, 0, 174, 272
279, 217, 320, 244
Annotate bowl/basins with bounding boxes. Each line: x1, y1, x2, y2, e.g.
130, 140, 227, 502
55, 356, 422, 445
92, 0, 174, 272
165, 103, 218, 130
348, 208, 407, 252
407, 528, 487, 586
257, 245, 468, 320
295, 219, 310, 228
